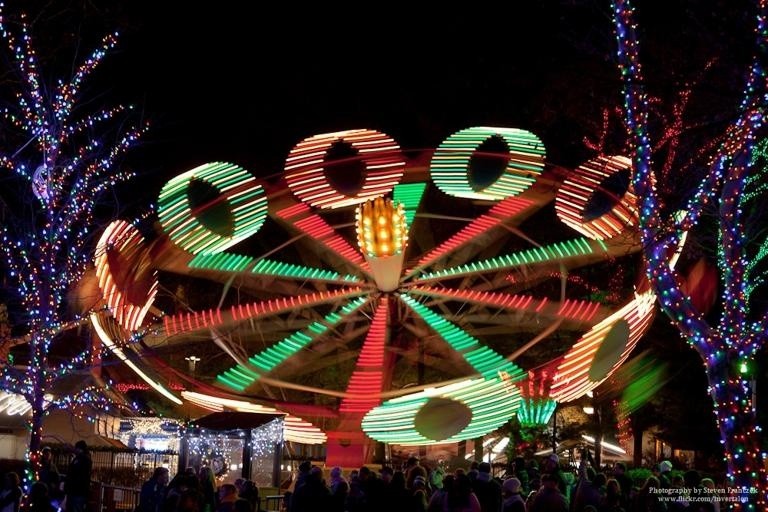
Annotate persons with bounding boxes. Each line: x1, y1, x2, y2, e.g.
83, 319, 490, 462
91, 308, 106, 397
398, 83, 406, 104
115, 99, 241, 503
1, 437, 731, 512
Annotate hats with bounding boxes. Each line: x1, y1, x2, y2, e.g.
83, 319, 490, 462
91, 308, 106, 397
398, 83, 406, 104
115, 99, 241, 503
299, 462, 312, 474
504, 478, 521, 492
550, 454, 559, 464
660, 461, 672, 473
